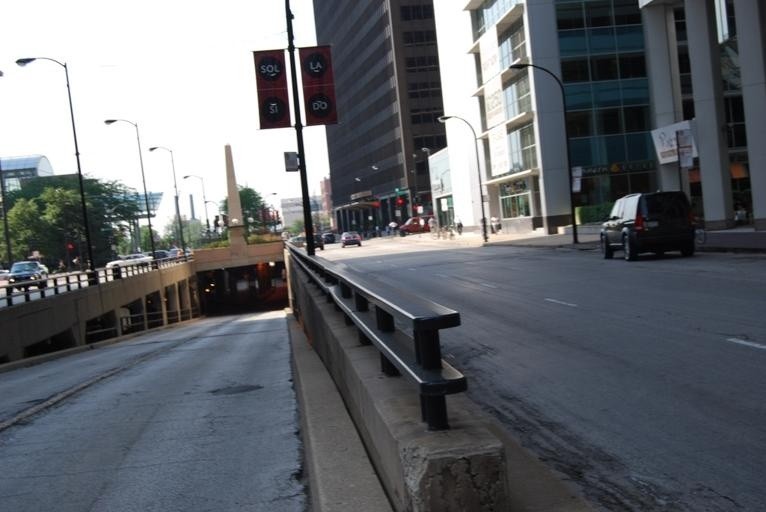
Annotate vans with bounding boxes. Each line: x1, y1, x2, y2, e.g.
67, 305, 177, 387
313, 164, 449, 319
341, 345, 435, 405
400, 215, 434, 237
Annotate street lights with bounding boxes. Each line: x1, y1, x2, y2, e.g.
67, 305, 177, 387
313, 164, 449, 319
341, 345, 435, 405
182, 172, 210, 238
204, 199, 224, 228
509, 61, 581, 246
262, 191, 278, 232
103, 119, 158, 269
149, 144, 188, 260
438, 114, 488, 243
13, 53, 99, 280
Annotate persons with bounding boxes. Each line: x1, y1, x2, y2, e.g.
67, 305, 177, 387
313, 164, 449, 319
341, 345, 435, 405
178, 249, 183, 262
454, 215, 463, 233
492, 215, 500, 232
689, 202, 702, 228
734, 200, 749, 224
428, 216, 438, 240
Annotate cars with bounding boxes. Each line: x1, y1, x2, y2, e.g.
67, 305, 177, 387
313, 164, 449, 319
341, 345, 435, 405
281, 231, 290, 239
0, 269, 10, 280
341, 231, 362, 247
185, 248, 193, 257
290, 235, 307, 247
322, 233, 336, 244
146, 295, 152, 304
153, 249, 170, 264
169, 249, 183, 259
106, 253, 154, 268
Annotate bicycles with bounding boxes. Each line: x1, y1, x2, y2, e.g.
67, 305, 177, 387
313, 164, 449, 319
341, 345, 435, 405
429, 223, 455, 241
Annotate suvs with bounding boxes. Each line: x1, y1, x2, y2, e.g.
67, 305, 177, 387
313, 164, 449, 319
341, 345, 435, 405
313, 232, 324, 250
598, 189, 697, 263
240, 269, 255, 281
7, 261, 49, 292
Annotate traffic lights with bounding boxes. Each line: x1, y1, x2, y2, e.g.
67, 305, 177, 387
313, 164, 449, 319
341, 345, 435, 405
416, 197, 422, 206
405, 193, 409, 204
